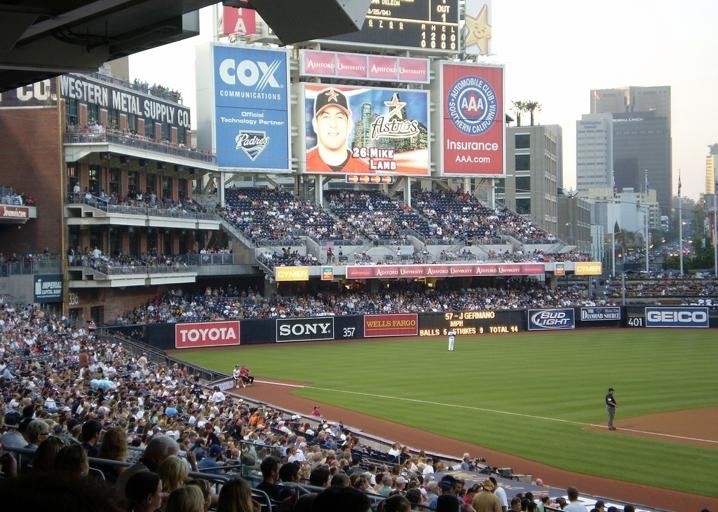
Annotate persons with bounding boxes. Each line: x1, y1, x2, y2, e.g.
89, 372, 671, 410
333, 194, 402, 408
306, 88, 375, 173
0, 290, 639, 512
0, 122, 716, 327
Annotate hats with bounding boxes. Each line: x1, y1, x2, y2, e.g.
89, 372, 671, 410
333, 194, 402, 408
315, 87, 350, 118
211, 444, 224, 453
480, 479, 494, 491
